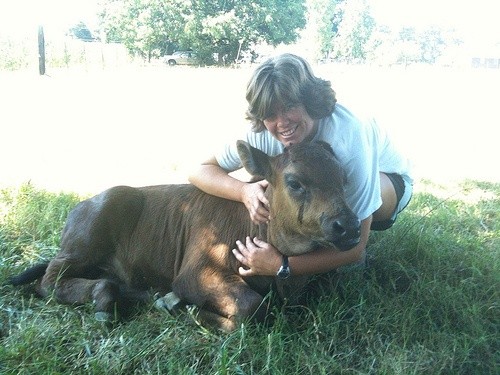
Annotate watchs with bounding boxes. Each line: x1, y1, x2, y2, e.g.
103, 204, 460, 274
277, 253, 291, 279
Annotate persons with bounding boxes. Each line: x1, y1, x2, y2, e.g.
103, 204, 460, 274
189, 52, 416, 278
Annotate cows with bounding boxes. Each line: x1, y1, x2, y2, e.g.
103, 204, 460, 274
8, 140, 362, 333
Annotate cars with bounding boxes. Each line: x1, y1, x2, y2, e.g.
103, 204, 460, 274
163, 51, 199, 66
